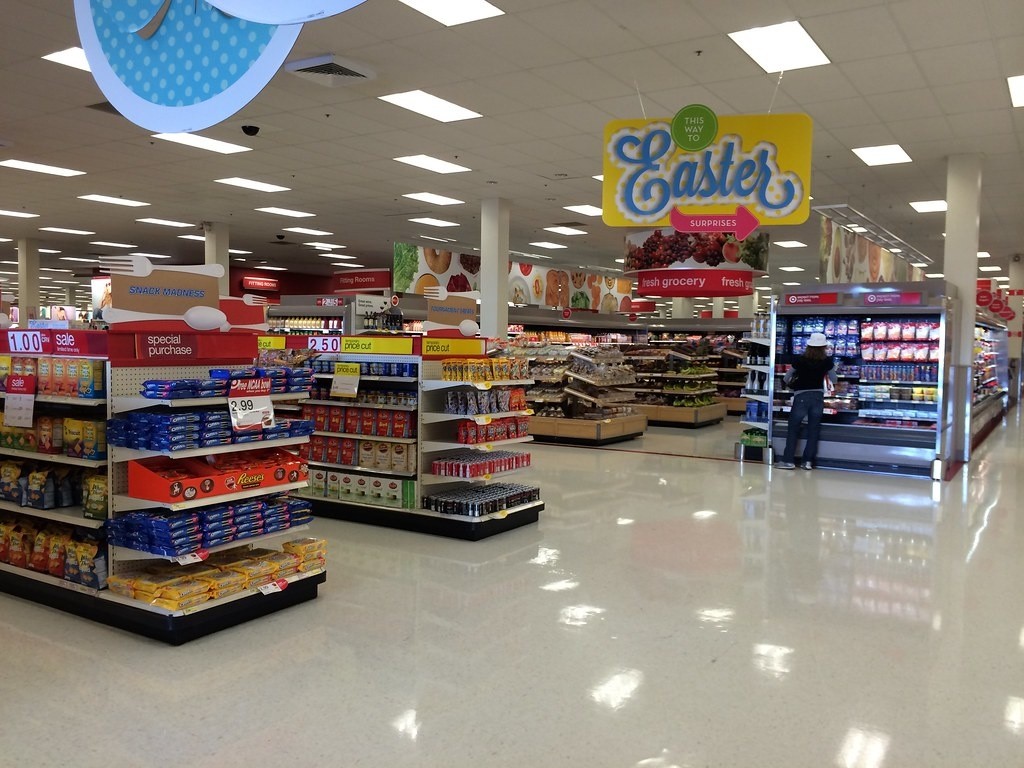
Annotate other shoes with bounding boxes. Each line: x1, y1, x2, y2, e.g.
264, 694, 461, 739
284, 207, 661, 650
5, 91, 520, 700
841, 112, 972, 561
775, 461, 795, 469
801, 461, 812, 470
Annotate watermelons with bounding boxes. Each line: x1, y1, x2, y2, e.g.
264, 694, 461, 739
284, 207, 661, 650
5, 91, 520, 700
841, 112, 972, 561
722, 242, 740, 263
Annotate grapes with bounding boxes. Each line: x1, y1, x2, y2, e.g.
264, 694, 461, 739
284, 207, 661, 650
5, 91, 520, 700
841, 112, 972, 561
643, 235, 721, 266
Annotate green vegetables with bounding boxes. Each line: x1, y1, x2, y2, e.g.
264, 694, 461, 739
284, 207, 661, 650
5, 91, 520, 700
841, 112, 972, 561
394, 242, 419, 293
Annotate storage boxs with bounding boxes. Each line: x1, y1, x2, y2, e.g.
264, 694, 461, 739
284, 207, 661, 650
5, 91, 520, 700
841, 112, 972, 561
1, 354, 108, 460
299, 360, 418, 510
128, 449, 309, 504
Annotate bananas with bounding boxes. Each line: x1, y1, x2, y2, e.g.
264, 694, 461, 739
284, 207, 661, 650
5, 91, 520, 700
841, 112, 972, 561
663, 365, 717, 406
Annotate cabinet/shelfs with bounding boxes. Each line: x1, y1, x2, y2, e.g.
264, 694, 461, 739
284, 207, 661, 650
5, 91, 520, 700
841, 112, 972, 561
419, 360, 546, 542
0, 354, 110, 626
274, 403, 302, 501
111, 366, 327, 645
298, 353, 419, 533
495, 322, 1001, 470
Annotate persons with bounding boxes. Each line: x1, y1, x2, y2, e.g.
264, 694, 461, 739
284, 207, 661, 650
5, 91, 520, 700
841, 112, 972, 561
59, 308, 67, 320
93, 281, 112, 319
773, 333, 835, 470
79, 311, 91, 323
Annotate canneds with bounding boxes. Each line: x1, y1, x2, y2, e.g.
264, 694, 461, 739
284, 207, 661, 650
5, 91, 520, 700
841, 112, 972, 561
433, 451, 530, 478
423, 481, 540, 516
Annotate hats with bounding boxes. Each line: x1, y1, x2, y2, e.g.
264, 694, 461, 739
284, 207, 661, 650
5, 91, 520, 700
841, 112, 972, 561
807, 333, 828, 347
